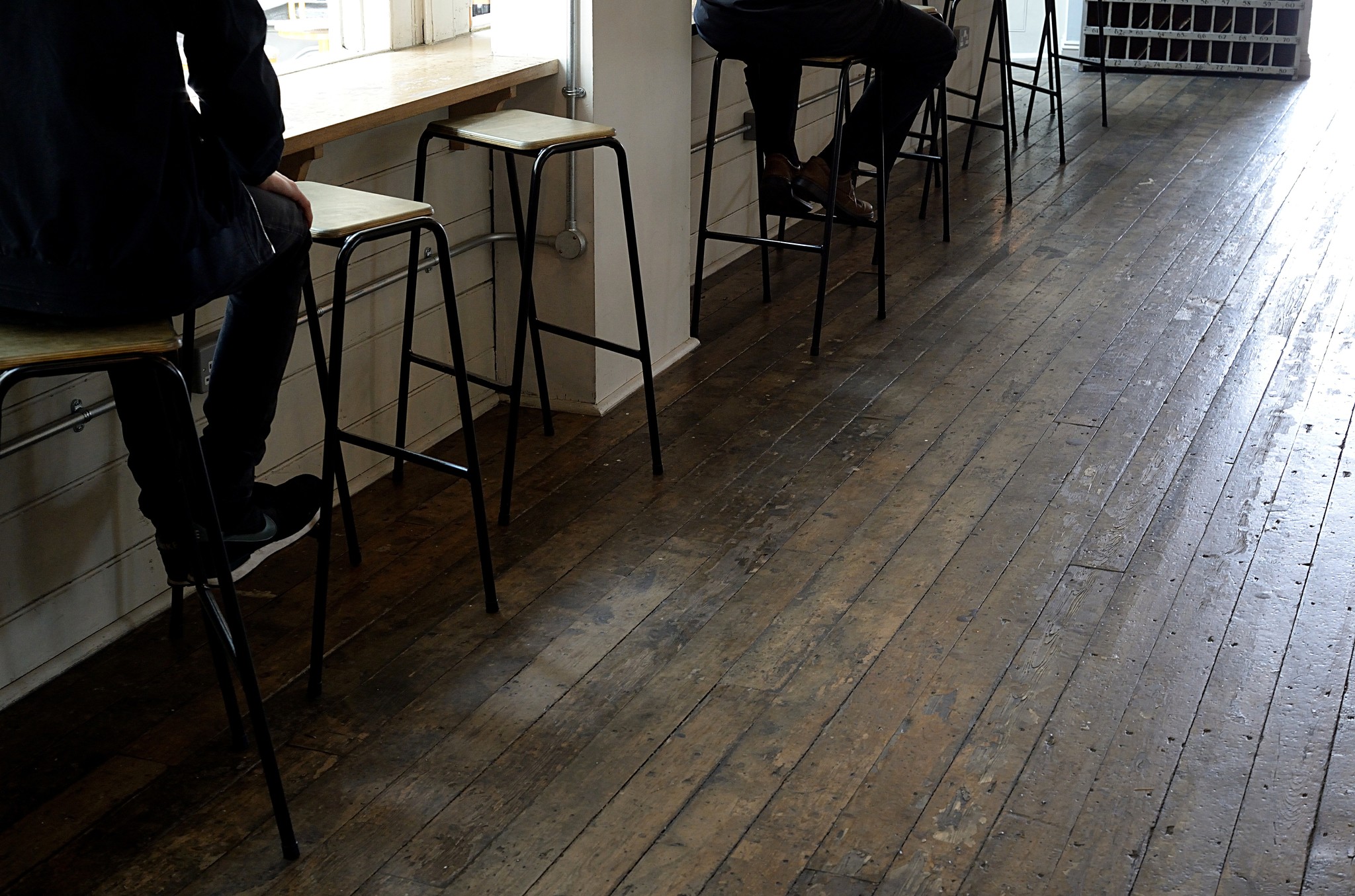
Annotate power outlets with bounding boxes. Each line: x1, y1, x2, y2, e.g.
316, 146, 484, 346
192, 329, 221, 394
743, 108, 757, 141
953, 26, 970, 51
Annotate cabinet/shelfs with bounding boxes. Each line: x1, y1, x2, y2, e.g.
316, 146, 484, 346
1078, 0, 1306, 81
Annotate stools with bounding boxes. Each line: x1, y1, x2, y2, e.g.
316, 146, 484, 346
0, 0, 1110, 866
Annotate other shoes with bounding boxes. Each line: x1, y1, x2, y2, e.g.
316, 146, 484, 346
153, 481, 275, 587
764, 154, 814, 211
791, 156, 874, 221
196, 473, 321, 586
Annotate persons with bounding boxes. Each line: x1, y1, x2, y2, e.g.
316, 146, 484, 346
0, 0, 324, 588
694, 0, 958, 221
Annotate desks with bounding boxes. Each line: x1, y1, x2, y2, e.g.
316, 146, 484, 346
177, 25, 558, 181
